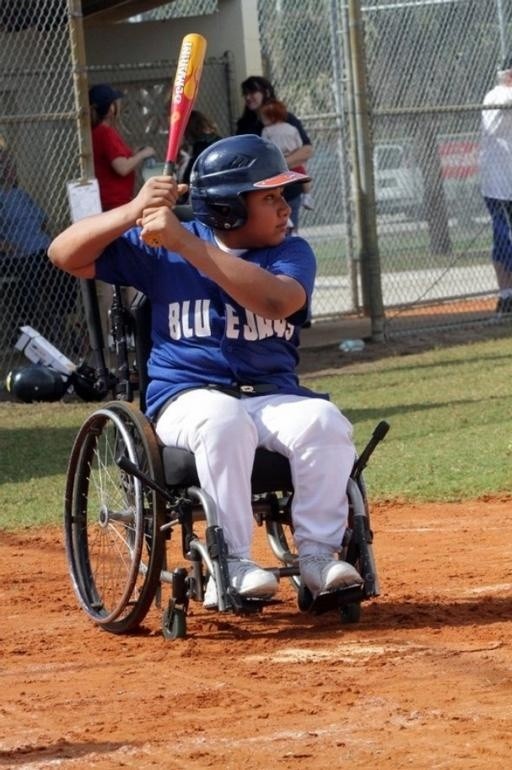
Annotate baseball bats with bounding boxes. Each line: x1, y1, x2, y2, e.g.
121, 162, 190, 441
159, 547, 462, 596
152, 33, 208, 247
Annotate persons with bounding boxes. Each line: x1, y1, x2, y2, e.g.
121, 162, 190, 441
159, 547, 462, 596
47, 129, 368, 620
175, 106, 223, 204
477, 48, 512, 316
234, 73, 315, 239
256, 96, 315, 211
86, 84, 158, 355
1, 149, 79, 317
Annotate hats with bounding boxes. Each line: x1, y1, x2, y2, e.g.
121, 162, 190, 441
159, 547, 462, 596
89, 84, 122, 104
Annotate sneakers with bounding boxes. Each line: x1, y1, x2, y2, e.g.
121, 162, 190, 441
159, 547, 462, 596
204, 560, 276, 610
495, 296, 512, 317
298, 556, 363, 595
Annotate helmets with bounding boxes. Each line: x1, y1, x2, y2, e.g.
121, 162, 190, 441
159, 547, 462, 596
6, 365, 65, 402
192, 135, 313, 231
71, 363, 119, 403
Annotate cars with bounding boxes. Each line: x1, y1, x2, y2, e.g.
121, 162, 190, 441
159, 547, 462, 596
371, 142, 423, 218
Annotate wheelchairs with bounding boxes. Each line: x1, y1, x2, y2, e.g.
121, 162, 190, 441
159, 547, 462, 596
42, 203, 391, 636
